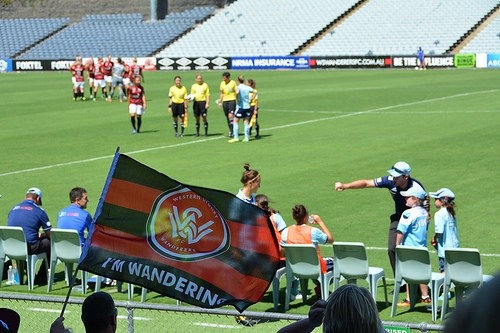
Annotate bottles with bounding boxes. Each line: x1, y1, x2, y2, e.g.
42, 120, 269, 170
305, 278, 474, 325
8, 266, 14, 285
13, 267, 19, 284
426, 304, 441, 311
308, 216, 317, 224
72, 285, 89, 291
47, 269, 50, 285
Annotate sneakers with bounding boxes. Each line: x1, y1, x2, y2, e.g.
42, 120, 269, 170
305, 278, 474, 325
228, 138, 239, 144
131, 128, 141, 134
65, 277, 79, 286
439, 292, 451, 300
255, 135, 260, 138
228, 132, 233, 137
72, 93, 130, 103
285, 288, 304, 299
196, 131, 208, 136
174, 133, 183, 138
242, 138, 249, 142
318, 290, 331, 300
236, 315, 261, 326
103, 278, 117, 286
37, 272, 58, 283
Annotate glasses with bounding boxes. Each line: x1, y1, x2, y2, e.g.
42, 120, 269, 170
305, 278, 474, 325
111, 306, 118, 316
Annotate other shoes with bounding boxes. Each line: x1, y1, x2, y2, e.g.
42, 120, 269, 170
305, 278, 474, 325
397, 299, 411, 307
399, 284, 406, 292
418, 297, 431, 303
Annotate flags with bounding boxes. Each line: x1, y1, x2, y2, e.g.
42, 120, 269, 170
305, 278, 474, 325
77, 154, 281, 314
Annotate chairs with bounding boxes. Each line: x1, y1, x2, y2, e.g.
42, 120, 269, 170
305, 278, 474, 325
273, 267, 286, 310
279, 243, 335, 310
390, 245, 450, 322
47, 227, 99, 295
440, 248, 495, 321
332, 241, 388, 309
94, 274, 182, 305
0, 225, 48, 289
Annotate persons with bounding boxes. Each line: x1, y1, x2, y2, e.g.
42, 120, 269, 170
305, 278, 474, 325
279, 205, 333, 297
441, 269, 500, 333
246, 79, 260, 138
168, 76, 190, 138
189, 75, 210, 137
396, 187, 431, 307
417, 46, 426, 70
276, 284, 388, 333
428, 188, 459, 300
71, 55, 144, 103
335, 162, 431, 292
228, 75, 257, 143
218, 72, 237, 137
8, 188, 52, 283
236, 164, 287, 232
0, 292, 117, 333
127, 76, 146, 134
57, 187, 93, 247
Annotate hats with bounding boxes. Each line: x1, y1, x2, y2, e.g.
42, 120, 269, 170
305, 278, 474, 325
427, 188, 456, 199
25, 187, 43, 206
386, 161, 411, 178
399, 186, 427, 199
